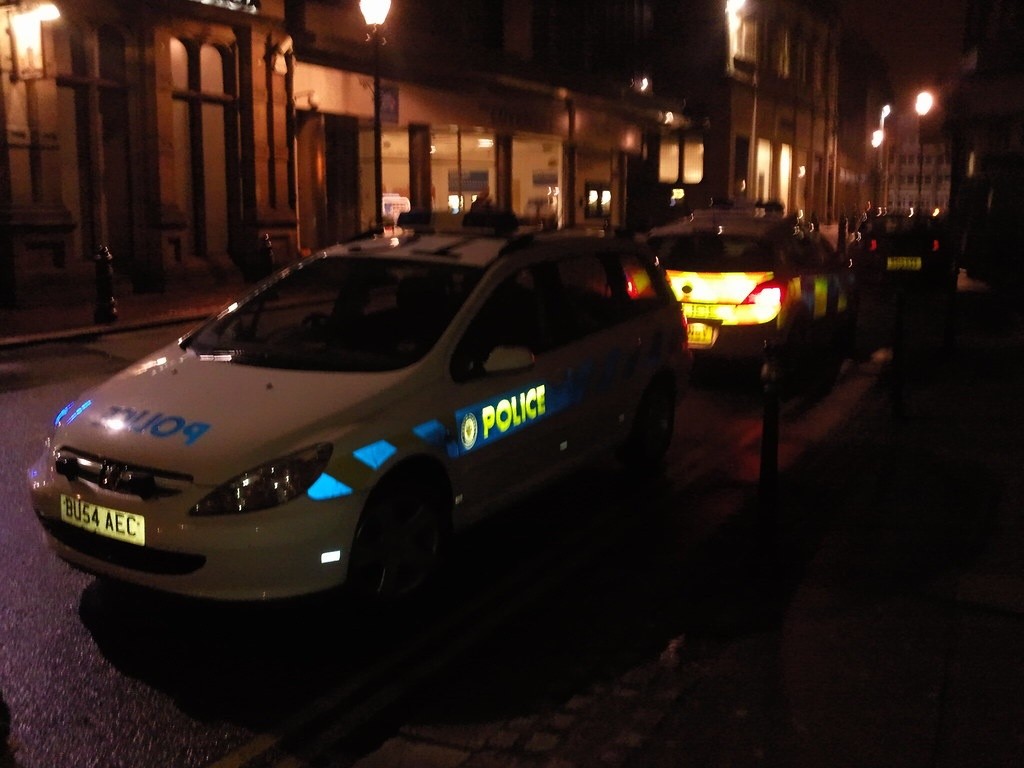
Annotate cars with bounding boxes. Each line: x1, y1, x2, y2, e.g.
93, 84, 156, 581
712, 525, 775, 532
647, 202, 856, 389
843, 212, 940, 295
27, 200, 696, 643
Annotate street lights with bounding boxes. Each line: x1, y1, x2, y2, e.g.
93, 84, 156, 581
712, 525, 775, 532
359, 0, 394, 235
914, 92, 935, 219
